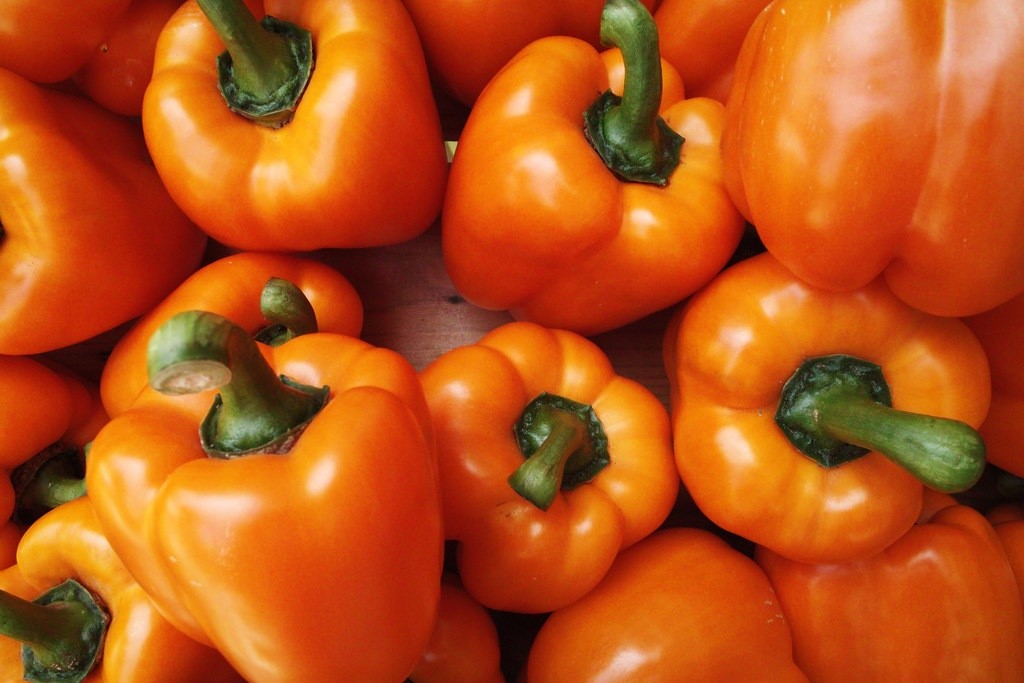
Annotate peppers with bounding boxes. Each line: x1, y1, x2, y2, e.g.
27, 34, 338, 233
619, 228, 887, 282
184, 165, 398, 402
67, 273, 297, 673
0, 0, 1024, 683
